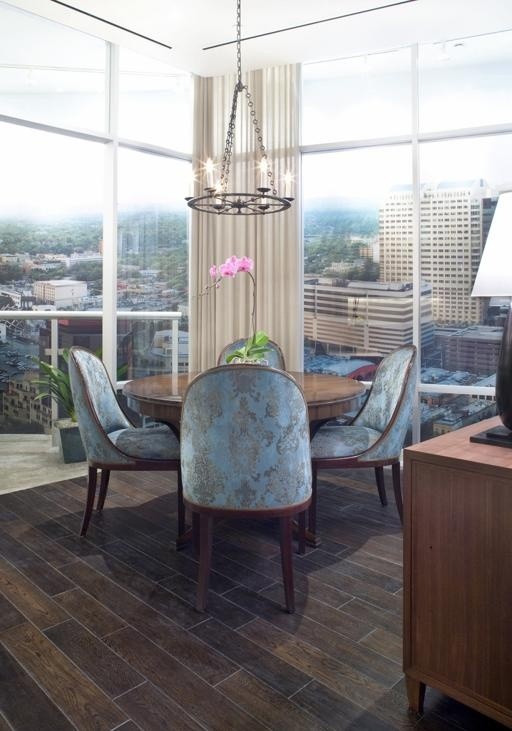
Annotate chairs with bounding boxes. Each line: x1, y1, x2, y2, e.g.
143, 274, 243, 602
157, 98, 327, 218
69, 344, 185, 550
180, 363, 314, 613
217, 337, 287, 374
305, 345, 419, 535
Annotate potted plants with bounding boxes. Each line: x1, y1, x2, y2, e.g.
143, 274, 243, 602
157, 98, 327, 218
23, 344, 130, 465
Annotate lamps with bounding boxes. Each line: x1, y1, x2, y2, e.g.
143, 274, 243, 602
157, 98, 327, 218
185, 1, 297, 218
469, 191, 511, 448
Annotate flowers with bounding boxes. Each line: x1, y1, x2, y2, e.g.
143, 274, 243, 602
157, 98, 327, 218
190, 255, 276, 368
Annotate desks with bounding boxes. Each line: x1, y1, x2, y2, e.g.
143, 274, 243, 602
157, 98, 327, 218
124, 370, 366, 559
